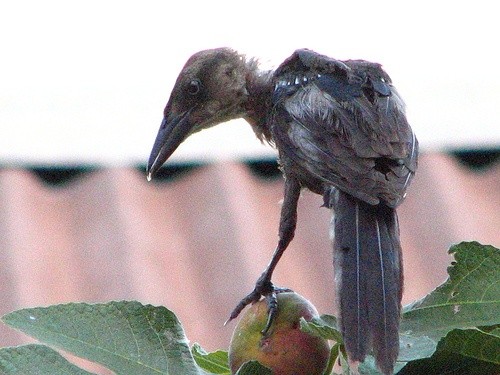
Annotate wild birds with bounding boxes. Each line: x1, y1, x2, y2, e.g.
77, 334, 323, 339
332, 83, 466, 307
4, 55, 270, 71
147, 47, 418, 375
